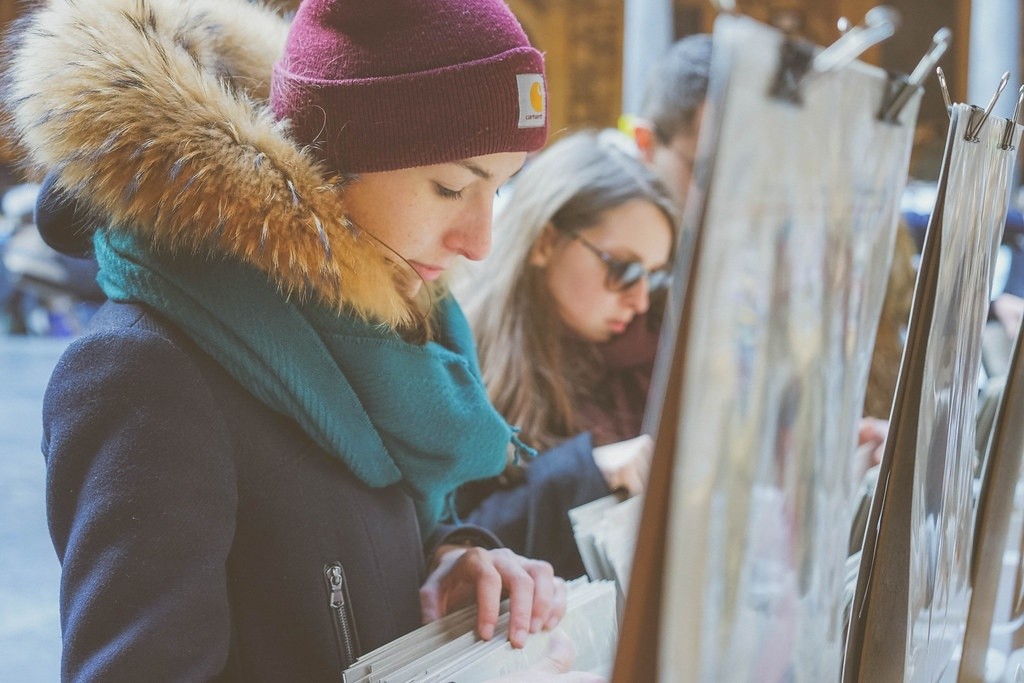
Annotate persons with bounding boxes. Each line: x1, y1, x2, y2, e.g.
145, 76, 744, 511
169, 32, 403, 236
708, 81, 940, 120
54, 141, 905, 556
0, 0, 608, 683
455, 32, 1024, 575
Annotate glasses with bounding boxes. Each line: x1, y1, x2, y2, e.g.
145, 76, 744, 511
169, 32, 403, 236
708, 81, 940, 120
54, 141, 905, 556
553, 217, 672, 296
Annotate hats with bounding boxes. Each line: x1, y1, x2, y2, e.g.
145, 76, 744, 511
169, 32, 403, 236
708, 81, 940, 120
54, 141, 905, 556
269, 1, 548, 174
